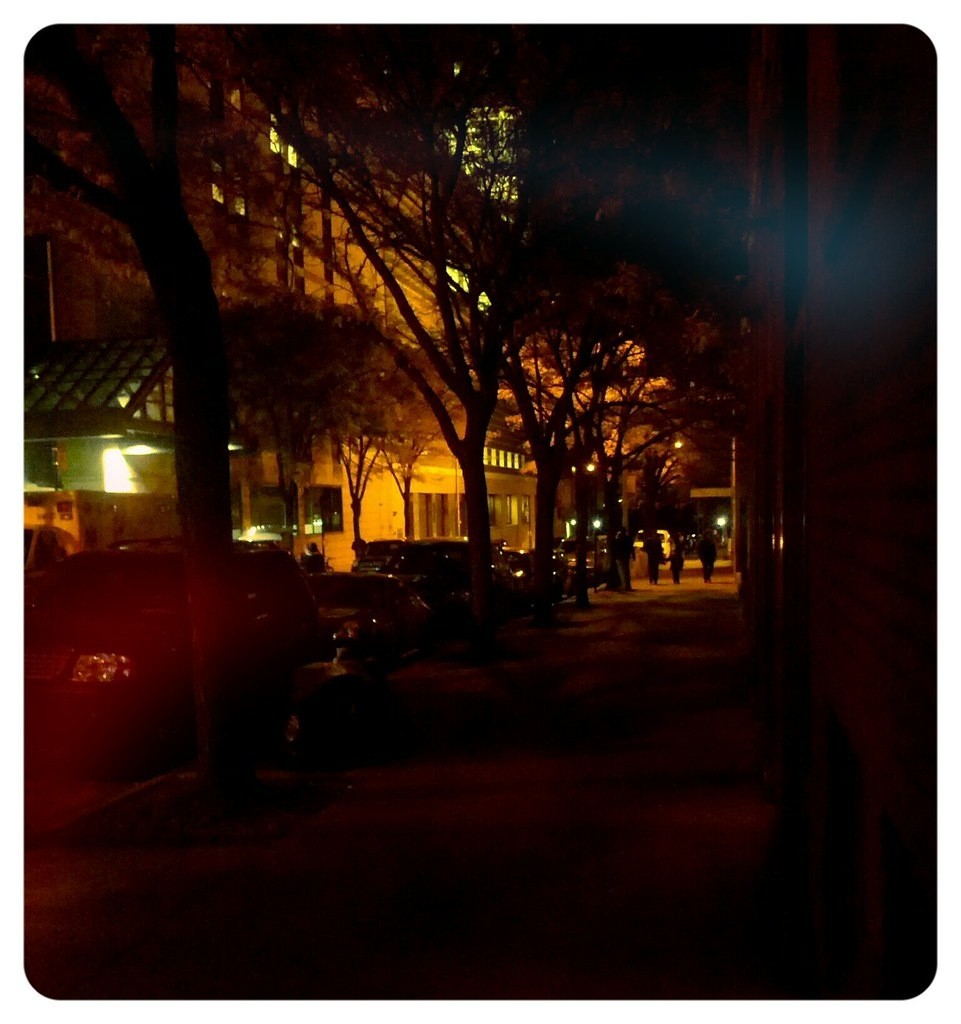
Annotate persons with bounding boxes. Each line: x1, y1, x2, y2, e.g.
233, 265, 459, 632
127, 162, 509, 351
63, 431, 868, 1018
648, 535, 668, 585
28, 513, 68, 565
300, 542, 325, 574
699, 529, 717, 584
614, 526, 637, 591
667, 548, 684, 585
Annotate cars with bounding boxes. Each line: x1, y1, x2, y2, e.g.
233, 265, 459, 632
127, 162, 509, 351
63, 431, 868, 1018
24, 534, 611, 781
